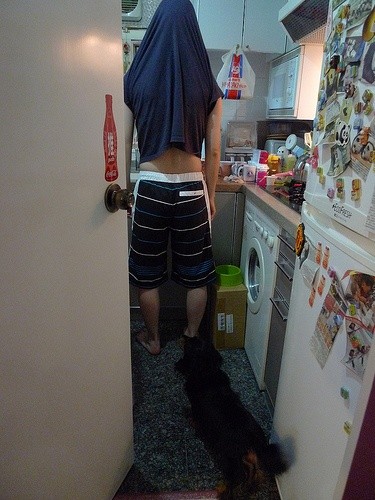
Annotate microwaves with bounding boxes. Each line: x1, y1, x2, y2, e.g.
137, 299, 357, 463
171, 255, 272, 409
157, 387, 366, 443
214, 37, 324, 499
266, 43, 323, 120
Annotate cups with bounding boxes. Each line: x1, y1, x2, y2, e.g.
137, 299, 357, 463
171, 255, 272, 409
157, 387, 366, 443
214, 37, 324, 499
231, 162, 247, 177
219, 161, 232, 178
238, 165, 256, 182
267, 176, 275, 186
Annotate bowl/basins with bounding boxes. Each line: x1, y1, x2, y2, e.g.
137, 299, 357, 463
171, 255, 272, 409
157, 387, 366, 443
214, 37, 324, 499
215, 265, 244, 286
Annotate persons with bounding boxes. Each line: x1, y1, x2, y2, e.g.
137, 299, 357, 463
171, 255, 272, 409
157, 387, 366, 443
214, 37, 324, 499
123, 0, 225, 355
351, 275, 375, 309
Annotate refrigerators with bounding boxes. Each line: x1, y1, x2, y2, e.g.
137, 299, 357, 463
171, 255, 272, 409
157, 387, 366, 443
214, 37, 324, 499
265, 0, 375, 500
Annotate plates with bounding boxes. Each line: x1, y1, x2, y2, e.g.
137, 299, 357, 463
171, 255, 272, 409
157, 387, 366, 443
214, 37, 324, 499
267, 134, 287, 139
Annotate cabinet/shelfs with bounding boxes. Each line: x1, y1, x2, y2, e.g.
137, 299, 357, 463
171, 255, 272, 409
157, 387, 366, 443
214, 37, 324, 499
130, 193, 244, 321
197, 0, 287, 54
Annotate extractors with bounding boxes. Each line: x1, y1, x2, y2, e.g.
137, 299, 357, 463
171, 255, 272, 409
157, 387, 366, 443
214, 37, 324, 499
276, 0, 328, 45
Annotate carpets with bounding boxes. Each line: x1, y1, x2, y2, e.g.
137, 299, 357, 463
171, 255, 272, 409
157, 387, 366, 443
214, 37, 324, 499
117, 320, 273, 493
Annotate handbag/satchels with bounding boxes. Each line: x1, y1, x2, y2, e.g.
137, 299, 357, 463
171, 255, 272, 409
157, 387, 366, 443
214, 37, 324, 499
216, 46, 255, 100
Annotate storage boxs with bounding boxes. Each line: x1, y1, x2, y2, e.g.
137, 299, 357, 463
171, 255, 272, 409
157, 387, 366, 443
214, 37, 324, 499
209, 282, 247, 350
